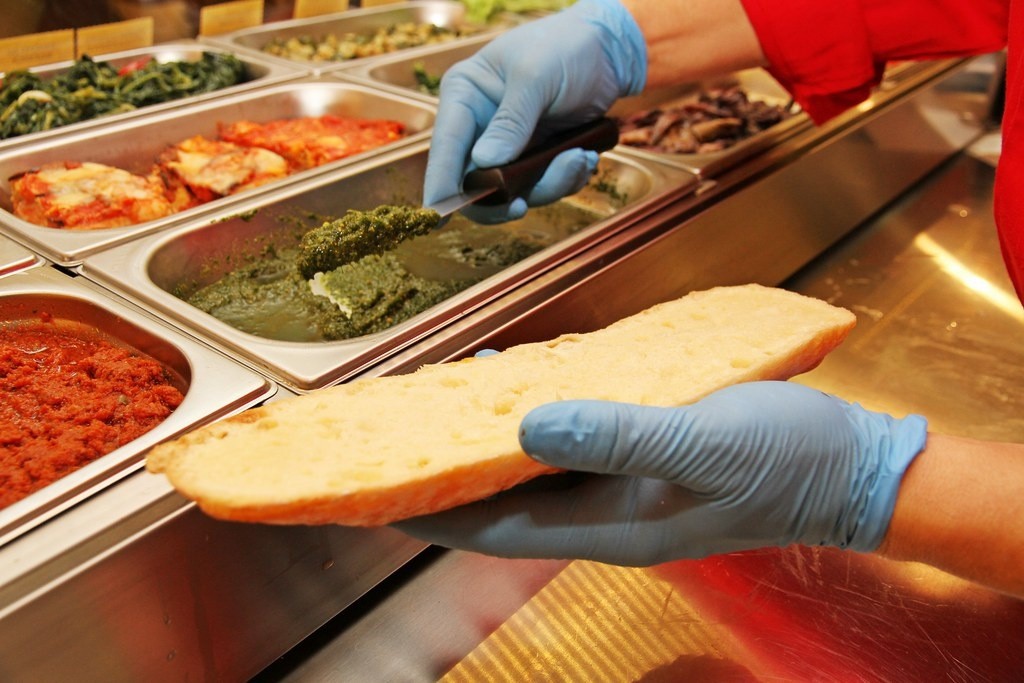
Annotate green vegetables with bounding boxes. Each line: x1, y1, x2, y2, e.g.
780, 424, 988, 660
410, 62, 441, 98
0, 53, 248, 141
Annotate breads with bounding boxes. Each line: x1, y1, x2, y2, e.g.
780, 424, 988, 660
143, 283, 855, 525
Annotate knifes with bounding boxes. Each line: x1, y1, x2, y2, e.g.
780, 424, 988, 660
418, 115, 622, 220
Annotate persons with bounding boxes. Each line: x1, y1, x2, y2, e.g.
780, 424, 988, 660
382, 0, 1024, 600
